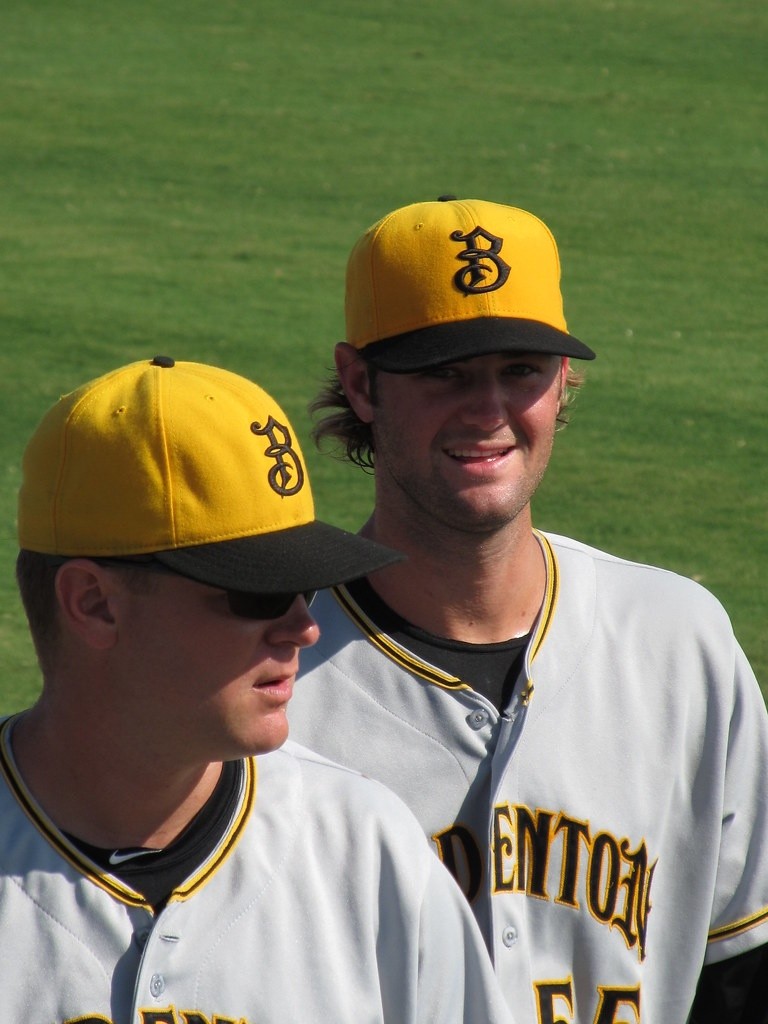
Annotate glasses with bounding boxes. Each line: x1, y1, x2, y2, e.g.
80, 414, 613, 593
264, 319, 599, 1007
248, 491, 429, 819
91, 555, 320, 622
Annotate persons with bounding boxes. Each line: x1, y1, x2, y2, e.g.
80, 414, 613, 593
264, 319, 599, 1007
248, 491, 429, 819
1, 356, 515, 1023
284, 192, 768, 1024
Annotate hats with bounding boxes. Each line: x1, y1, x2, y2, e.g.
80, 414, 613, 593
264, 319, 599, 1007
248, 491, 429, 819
18, 356, 408, 596
344, 194, 596, 373
